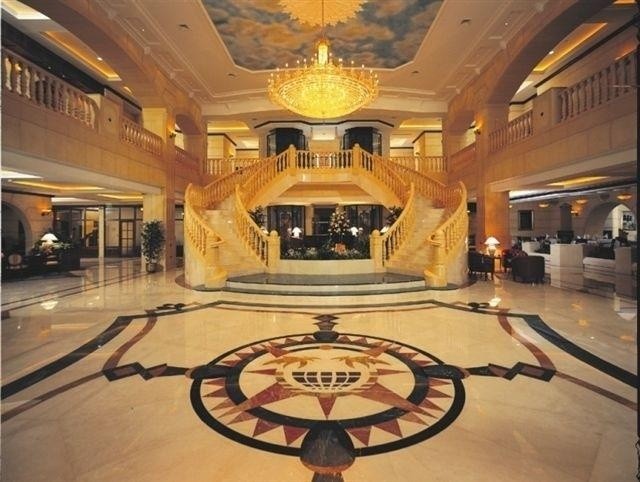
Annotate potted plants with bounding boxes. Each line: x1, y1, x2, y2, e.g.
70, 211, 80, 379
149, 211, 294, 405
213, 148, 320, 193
140, 220, 166, 272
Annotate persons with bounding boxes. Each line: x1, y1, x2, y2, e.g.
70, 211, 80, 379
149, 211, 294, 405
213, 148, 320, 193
287, 224, 302, 237
350, 224, 363, 236
380, 223, 390, 233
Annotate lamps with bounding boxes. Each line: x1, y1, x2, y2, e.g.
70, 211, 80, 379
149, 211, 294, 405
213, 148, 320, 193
483, 237, 500, 257
264, 0, 380, 119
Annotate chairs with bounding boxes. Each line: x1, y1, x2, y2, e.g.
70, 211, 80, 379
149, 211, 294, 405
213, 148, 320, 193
468, 251, 545, 282
1, 246, 80, 280
